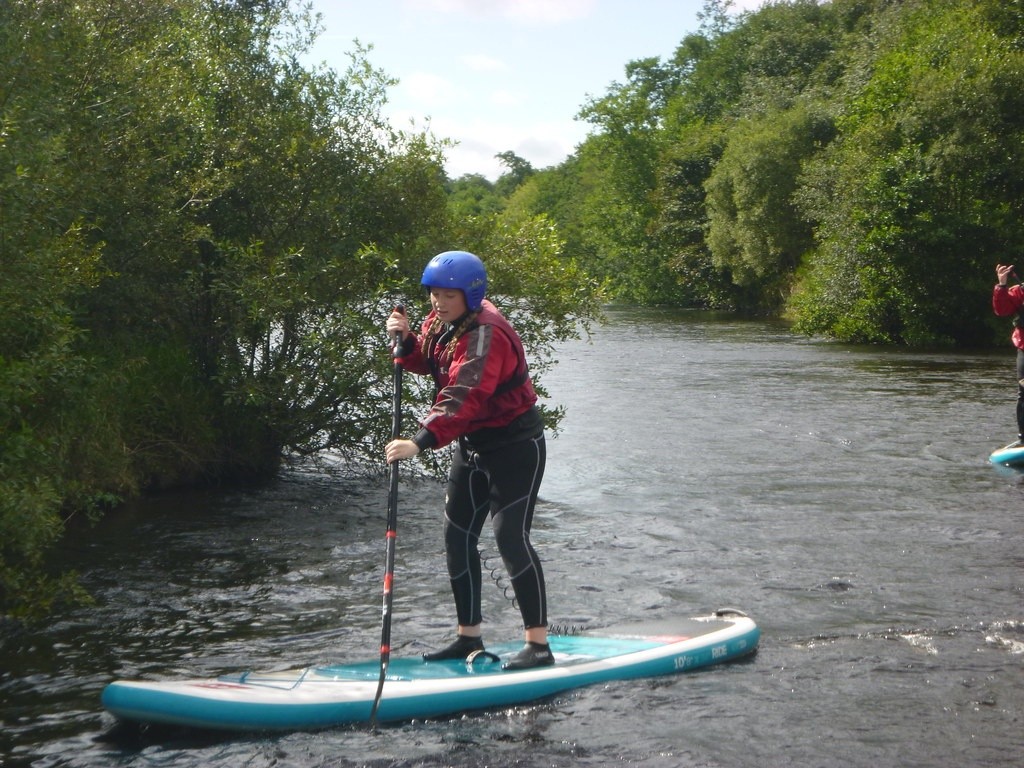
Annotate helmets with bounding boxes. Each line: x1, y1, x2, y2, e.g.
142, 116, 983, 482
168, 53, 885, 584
421, 251, 486, 312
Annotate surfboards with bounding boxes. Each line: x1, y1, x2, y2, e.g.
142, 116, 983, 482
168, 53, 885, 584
101, 605, 759, 733
989, 439, 1024, 464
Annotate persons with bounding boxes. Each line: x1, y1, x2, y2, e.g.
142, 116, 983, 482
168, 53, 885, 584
386, 249, 558, 669
992, 261, 1023, 446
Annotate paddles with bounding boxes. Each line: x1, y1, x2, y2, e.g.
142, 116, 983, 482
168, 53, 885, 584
363, 296, 406, 733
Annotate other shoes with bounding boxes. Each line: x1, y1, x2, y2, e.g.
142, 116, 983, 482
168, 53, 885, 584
501, 640, 555, 669
423, 635, 485, 662
